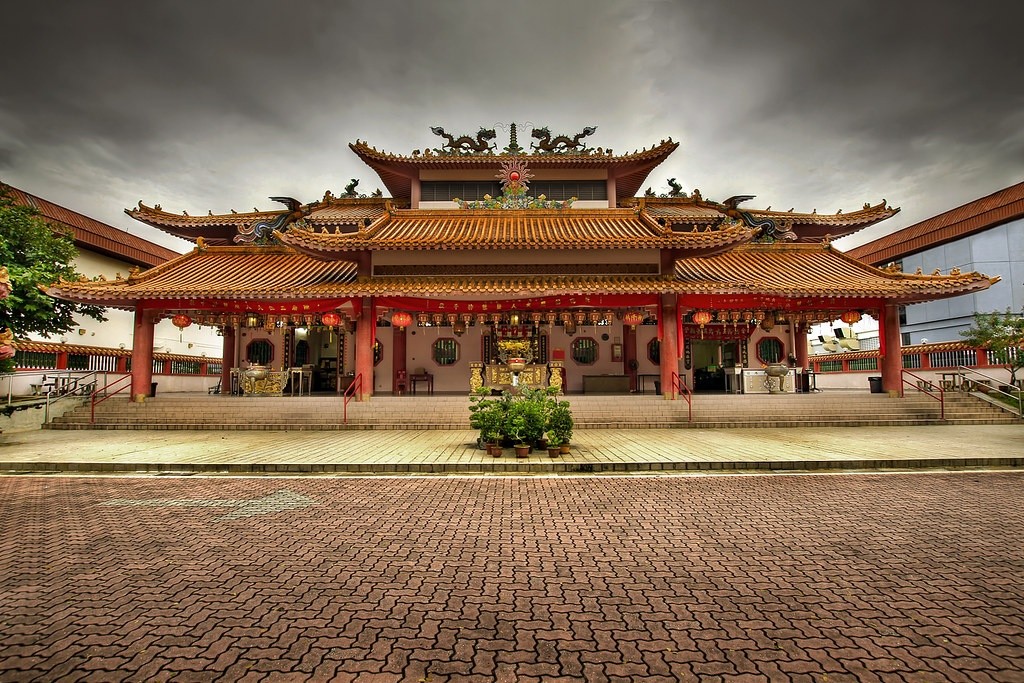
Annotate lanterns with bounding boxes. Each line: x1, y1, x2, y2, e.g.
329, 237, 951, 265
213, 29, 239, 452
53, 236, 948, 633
717, 310, 837, 332
692, 310, 711, 339
391, 310, 413, 332
622, 307, 644, 331
321, 312, 341, 344
841, 309, 861, 328
172, 313, 192, 343
418, 311, 527, 336
196, 313, 312, 340
529, 310, 615, 336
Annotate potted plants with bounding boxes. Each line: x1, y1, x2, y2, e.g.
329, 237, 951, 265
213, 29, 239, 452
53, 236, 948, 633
468, 383, 574, 458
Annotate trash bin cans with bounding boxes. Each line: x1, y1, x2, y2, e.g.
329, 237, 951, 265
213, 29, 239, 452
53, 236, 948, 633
151, 382, 158, 397
868, 377, 882, 393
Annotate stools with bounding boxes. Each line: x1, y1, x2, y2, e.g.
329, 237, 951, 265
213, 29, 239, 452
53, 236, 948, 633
44, 383, 55, 396
208, 385, 222, 393
971, 379, 991, 391
30, 384, 42, 396
916, 380, 933, 391
79, 384, 98, 395
939, 379, 953, 391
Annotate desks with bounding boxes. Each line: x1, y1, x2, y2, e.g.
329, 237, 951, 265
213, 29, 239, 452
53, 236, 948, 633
238, 368, 291, 397
469, 360, 483, 395
409, 374, 433, 396
339, 375, 375, 394
549, 361, 564, 396
638, 373, 687, 396
48, 376, 85, 396
935, 372, 972, 392
291, 367, 312, 396
229, 368, 246, 397
722, 366, 743, 395
483, 361, 549, 394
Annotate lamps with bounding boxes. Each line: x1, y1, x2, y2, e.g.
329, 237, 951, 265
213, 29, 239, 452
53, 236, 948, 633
843, 347, 848, 352
921, 337, 928, 344
60, 336, 69, 343
201, 352, 207, 357
118, 343, 126, 349
510, 310, 519, 328
165, 348, 172, 353
